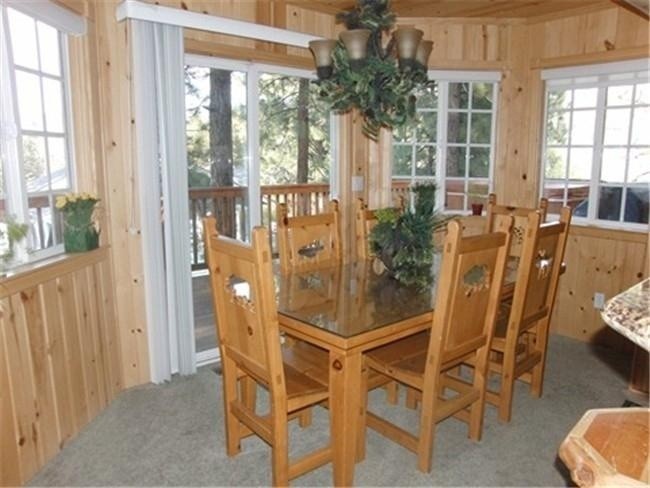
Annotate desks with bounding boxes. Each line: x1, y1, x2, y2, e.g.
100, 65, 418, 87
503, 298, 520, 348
555, 276, 648, 488
222, 253, 567, 487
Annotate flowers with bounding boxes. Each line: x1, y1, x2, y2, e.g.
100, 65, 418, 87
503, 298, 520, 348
54, 189, 97, 213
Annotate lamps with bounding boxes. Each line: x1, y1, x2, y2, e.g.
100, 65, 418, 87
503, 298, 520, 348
302, 0, 437, 135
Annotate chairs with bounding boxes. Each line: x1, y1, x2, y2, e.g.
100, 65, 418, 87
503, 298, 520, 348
355, 214, 515, 472
355, 206, 404, 259
277, 199, 398, 425
438, 206, 572, 425
483, 193, 549, 362
203, 214, 362, 487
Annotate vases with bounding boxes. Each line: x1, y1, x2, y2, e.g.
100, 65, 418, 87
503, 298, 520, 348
65, 216, 101, 249
